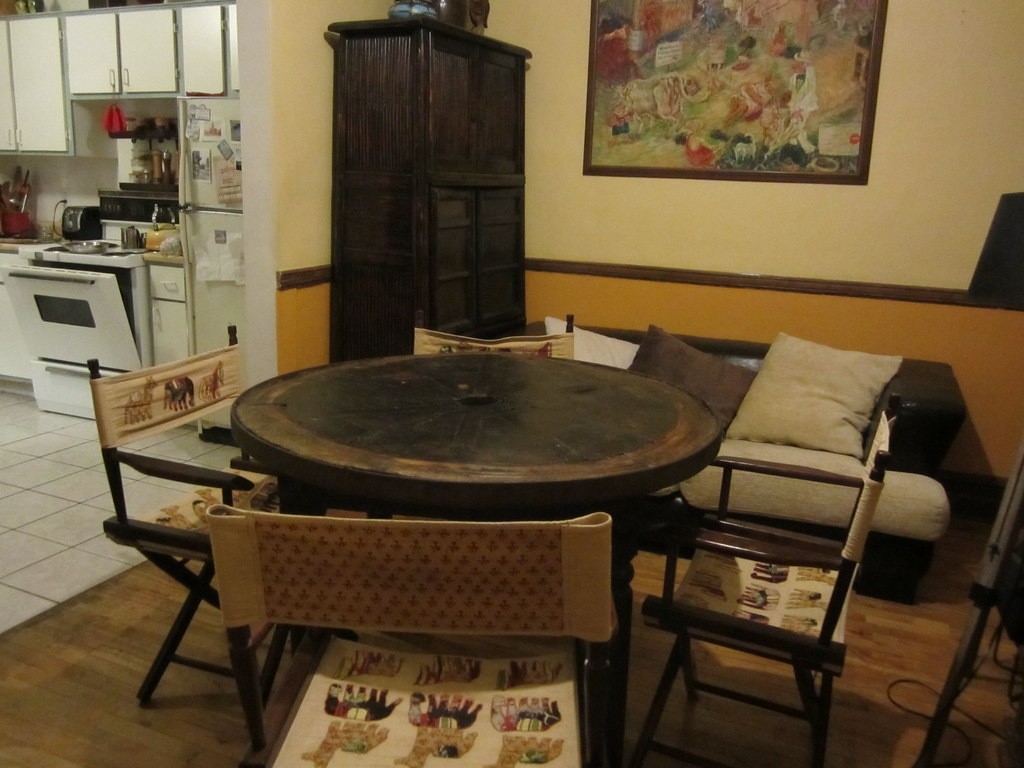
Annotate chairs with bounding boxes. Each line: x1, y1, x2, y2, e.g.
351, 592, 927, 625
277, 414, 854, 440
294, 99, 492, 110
86, 327, 330, 711
629, 393, 900, 768
207, 502, 625, 767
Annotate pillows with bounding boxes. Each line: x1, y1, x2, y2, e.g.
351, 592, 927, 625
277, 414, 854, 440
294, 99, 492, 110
542, 315, 639, 371
620, 327, 759, 435
722, 332, 905, 458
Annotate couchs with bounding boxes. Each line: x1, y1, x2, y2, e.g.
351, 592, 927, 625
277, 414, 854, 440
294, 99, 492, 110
513, 319, 969, 605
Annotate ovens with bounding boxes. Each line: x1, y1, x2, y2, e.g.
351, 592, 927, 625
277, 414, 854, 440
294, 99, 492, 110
0, 266, 155, 420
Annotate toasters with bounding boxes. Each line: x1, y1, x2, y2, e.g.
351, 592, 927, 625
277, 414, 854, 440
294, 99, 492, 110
62, 205, 101, 242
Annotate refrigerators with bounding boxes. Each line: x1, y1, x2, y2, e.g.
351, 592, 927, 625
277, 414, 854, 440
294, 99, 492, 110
176, 96, 246, 430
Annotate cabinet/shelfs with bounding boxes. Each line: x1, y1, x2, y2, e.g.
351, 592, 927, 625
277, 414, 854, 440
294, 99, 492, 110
328, 16, 535, 360
148, 263, 189, 365
1, 0, 242, 157
0, 250, 35, 398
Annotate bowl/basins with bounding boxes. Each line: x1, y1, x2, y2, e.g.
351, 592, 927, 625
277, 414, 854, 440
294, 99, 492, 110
65, 242, 109, 254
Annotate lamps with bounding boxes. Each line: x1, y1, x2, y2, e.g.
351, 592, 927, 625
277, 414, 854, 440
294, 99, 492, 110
969, 190, 1023, 609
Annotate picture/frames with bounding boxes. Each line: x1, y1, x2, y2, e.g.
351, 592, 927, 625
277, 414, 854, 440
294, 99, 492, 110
584, 0, 890, 187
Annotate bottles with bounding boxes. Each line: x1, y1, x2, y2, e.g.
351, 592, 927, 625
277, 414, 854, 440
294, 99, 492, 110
126, 226, 141, 250
124, 117, 137, 131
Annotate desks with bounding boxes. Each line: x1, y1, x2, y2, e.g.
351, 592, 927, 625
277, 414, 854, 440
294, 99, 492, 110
228, 355, 722, 758
